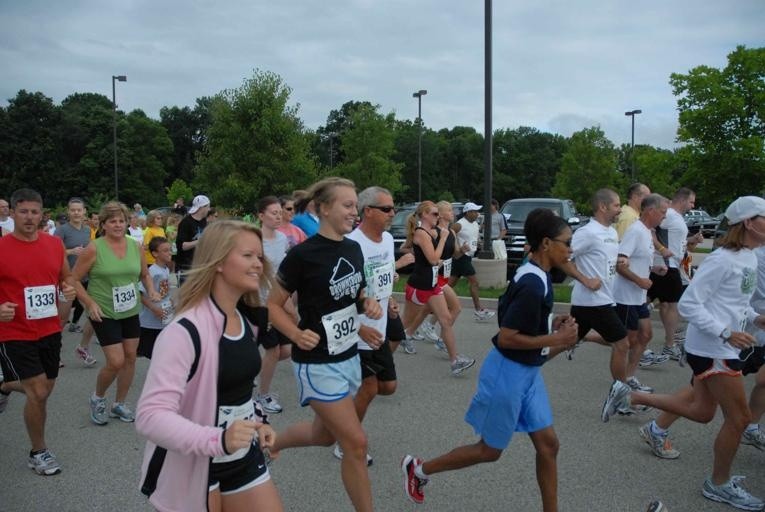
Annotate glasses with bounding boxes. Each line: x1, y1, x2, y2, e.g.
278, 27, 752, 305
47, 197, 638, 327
368, 205, 395, 213
551, 237, 572, 248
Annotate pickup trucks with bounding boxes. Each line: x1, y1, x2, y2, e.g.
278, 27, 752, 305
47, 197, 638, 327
481, 197, 591, 273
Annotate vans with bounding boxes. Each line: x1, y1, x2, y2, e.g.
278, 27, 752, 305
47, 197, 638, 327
685, 209, 711, 222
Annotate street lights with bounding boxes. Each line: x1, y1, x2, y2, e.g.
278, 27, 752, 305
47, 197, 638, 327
412, 90, 428, 201
325, 130, 338, 170
112, 75, 127, 200
625, 109, 642, 184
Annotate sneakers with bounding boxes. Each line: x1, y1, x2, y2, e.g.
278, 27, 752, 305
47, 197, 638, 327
89, 393, 109, 425
741, 429, 765, 450
400, 320, 448, 354
601, 346, 682, 459
110, 401, 135, 422
257, 393, 282, 413
76, 346, 96, 365
703, 479, 765, 512
334, 443, 373, 467
28, 447, 62, 475
400, 454, 428, 504
451, 357, 475, 375
68, 323, 83, 332
472, 308, 496, 321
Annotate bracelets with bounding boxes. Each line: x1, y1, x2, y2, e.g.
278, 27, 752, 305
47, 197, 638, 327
659, 246, 664, 251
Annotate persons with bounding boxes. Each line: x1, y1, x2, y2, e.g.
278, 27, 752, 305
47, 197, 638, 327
237, 190, 321, 412
1, 189, 77, 475
400, 208, 578, 512
135, 221, 284, 511
266, 178, 383, 511
75, 202, 160, 425
335, 187, 398, 467
561, 183, 704, 393
387, 200, 505, 375
638, 247, 764, 459
1, 194, 218, 368
602, 195, 764, 511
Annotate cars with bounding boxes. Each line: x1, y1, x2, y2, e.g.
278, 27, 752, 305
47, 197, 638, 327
389, 200, 484, 252
156, 206, 191, 216
683, 215, 721, 237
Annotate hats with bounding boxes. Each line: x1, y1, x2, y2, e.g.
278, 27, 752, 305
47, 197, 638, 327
463, 202, 483, 213
188, 195, 209, 214
725, 196, 765, 225
